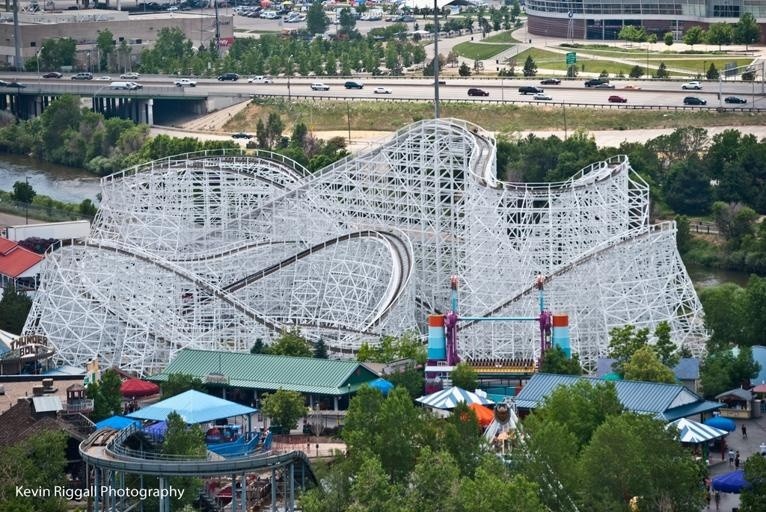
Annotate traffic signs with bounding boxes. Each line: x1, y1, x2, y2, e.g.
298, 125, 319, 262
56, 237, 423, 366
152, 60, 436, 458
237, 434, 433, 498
566, 52, 577, 64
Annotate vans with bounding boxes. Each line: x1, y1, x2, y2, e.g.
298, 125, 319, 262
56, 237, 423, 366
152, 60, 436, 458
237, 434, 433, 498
684, 97, 706, 105
344, 81, 362, 89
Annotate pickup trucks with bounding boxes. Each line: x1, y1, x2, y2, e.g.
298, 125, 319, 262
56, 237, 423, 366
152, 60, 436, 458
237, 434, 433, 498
248, 76, 273, 83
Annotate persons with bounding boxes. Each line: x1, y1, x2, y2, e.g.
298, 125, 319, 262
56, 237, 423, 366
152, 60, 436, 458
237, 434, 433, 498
728, 450, 735, 464
741, 424, 747, 438
735, 451, 740, 467
262, 429, 268, 443
706, 477, 721, 510
760, 442, 766, 455
124, 399, 138, 415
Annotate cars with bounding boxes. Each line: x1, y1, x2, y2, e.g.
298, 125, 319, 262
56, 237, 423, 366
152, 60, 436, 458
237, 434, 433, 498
174, 79, 197, 87
43, 72, 62, 78
361, 15, 380, 21
434, 81, 447, 85
609, 96, 627, 102
585, 79, 641, 90
71, 71, 142, 92
167, 6, 178, 11
232, 134, 250, 139
236, 6, 306, 22
0, 80, 27, 88
725, 97, 747, 104
519, 79, 561, 100
385, 15, 417, 22
374, 88, 392, 94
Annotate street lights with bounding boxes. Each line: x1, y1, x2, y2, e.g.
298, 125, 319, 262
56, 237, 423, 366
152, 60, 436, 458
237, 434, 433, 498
37, 47, 45, 80
25, 176, 32, 225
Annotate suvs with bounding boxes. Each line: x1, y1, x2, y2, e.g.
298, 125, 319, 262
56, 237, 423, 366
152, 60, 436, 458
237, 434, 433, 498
218, 73, 239, 81
681, 81, 703, 90
311, 82, 329, 91
468, 89, 490, 96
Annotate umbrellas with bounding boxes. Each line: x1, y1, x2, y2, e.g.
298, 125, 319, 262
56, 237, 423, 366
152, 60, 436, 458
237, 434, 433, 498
119, 379, 159, 396
712, 469, 753, 494
704, 417, 736, 432
